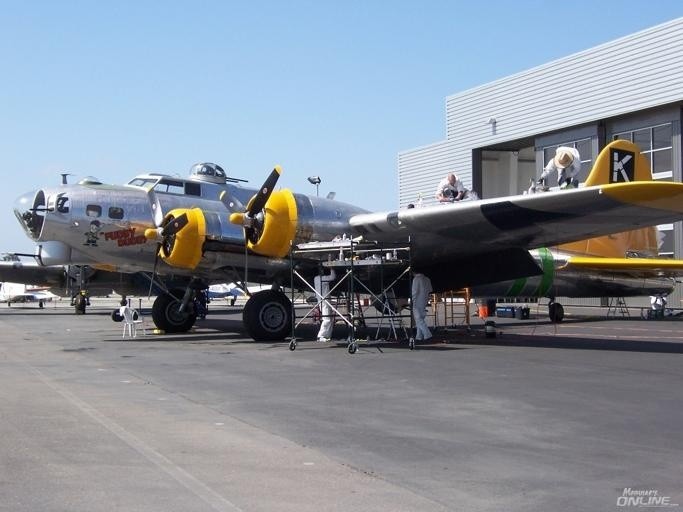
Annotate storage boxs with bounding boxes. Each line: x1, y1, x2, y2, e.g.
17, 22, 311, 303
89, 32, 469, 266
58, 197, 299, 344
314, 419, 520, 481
495, 307, 515, 318
514, 307, 530, 320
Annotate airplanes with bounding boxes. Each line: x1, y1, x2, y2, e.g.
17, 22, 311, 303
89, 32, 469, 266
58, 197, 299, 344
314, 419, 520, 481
0, 139, 683, 342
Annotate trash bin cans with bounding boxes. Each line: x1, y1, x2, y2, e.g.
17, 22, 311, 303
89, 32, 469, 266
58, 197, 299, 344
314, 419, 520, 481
497, 307, 530, 320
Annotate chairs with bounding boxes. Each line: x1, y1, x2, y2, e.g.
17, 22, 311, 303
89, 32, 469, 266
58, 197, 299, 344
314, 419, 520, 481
119, 306, 146, 338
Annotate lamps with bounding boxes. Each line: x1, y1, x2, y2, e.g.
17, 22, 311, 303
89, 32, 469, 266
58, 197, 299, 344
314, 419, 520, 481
486, 116, 496, 125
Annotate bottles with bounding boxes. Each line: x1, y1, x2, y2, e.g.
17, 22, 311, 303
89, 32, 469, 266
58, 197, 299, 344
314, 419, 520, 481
338, 247, 344, 261
392, 249, 397, 260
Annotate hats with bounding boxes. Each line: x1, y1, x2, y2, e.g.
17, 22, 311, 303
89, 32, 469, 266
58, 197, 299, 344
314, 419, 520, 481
555, 150, 574, 168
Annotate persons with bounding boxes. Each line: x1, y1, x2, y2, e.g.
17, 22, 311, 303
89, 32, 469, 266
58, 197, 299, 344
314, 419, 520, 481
535, 145, 581, 190
649, 296, 666, 309
411, 269, 433, 343
435, 173, 468, 203
313, 268, 335, 342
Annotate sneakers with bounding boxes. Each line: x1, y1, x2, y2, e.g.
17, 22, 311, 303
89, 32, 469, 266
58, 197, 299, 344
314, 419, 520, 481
415, 335, 432, 340
317, 336, 330, 342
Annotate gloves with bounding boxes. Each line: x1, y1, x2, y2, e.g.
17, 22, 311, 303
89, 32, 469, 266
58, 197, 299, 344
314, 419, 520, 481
538, 179, 543, 184
